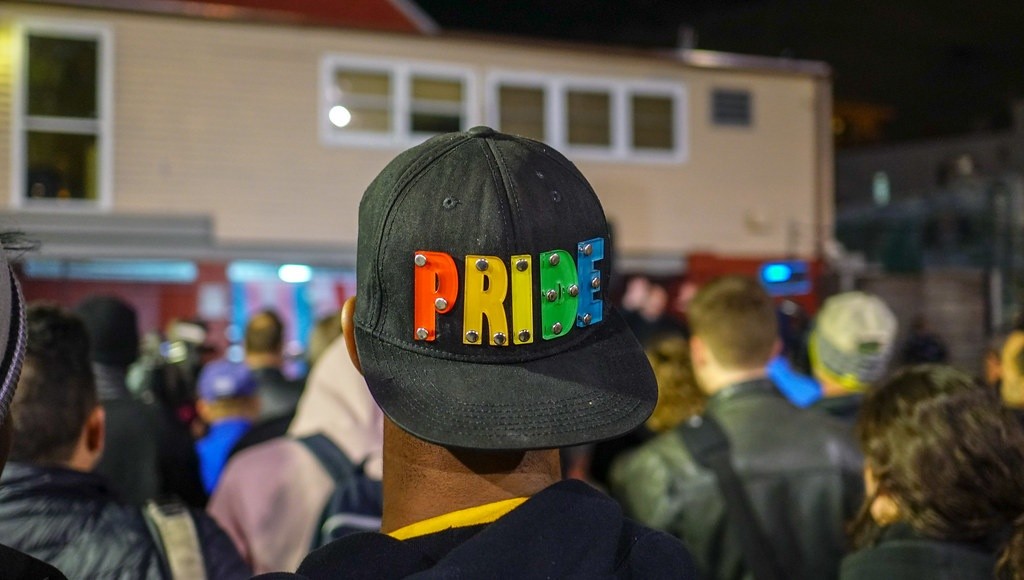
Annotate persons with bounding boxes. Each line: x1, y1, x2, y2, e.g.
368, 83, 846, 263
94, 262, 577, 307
73, 292, 212, 518
204, 330, 383, 573
836, 363, 1024, 580
194, 359, 260, 493
0, 301, 174, 580
0, 257, 1024, 483
611, 274, 864, 579
247, 123, 705, 579
804, 292, 900, 425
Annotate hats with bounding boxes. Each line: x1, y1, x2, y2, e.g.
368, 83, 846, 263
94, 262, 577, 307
66, 295, 142, 367
348, 126, 661, 450
1, 247, 30, 421
195, 358, 260, 403
812, 289, 898, 382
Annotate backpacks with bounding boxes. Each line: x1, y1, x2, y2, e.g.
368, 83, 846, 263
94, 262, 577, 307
301, 432, 382, 551
